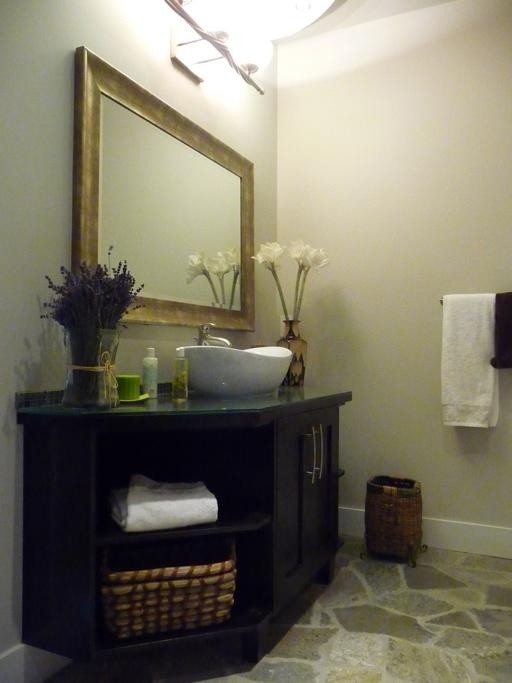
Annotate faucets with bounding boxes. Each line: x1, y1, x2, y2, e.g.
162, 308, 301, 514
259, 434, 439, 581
193, 322, 232, 348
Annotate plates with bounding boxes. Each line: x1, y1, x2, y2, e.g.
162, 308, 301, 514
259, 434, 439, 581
114, 393, 150, 404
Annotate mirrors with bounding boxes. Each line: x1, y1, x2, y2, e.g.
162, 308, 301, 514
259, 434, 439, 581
71, 46, 259, 331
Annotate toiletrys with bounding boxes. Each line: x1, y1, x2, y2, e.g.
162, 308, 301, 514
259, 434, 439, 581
115, 375, 140, 400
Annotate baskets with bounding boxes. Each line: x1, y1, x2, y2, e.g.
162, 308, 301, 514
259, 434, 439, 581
102, 542, 236, 639
365, 475, 423, 557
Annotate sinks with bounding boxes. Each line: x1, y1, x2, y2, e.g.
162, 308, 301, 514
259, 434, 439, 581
177, 346, 293, 396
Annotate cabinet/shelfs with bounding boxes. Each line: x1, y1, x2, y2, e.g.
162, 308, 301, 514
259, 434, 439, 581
277, 399, 337, 593
16, 387, 353, 672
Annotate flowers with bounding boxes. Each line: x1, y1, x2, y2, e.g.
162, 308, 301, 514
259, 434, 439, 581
249, 241, 326, 319
37, 244, 148, 328
183, 249, 242, 309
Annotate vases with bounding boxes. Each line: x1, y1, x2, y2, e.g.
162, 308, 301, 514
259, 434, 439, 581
278, 316, 305, 387
59, 325, 123, 408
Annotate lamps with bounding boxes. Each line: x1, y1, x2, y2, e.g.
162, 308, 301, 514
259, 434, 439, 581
164, 0, 274, 96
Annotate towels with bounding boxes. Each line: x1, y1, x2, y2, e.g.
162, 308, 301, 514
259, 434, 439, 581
440, 288, 501, 431
491, 295, 511, 366
111, 474, 222, 530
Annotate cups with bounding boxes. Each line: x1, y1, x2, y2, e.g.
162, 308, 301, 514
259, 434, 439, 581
116, 375, 143, 400
